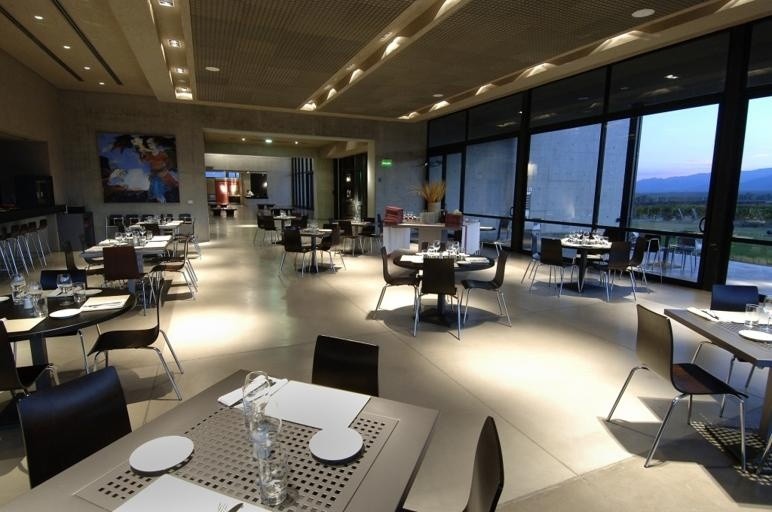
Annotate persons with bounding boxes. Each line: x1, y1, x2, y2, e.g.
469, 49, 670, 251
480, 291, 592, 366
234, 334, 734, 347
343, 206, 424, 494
246, 190, 255, 197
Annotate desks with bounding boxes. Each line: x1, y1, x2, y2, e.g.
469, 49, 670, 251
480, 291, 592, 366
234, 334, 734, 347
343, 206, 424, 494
0, 370, 440, 511
392, 250, 495, 328
664, 308, 770, 474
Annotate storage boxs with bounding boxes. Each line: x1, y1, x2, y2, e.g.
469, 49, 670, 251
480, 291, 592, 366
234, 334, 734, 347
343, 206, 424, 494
210, 207, 237, 217
270, 207, 294, 216
256, 202, 276, 210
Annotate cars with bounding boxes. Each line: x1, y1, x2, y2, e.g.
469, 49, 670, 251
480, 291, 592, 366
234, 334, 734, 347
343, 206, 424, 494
567, 229, 609, 246
744, 304, 759, 326
32, 291, 49, 317
280, 210, 287, 218
306, 220, 320, 232
247, 419, 291, 505
415, 240, 467, 263
72, 282, 87, 303
144, 215, 172, 225
402, 210, 416, 222
99, 226, 152, 247
352, 217, 361, 223
242, 372, 269, 430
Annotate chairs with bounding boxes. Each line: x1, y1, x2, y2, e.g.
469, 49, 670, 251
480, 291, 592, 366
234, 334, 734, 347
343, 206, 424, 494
401, 416, 505, 512
373, 246, 422, 325
521, 226, 697, 302
253, 213, 383, 280
607, 305, 748, 472
1, 213, 202, 400
690, 285, 759, 417
312, 335, 379, 396
413, 257, 460, 339
460, 245, 511, 326
15, 366, 133, 490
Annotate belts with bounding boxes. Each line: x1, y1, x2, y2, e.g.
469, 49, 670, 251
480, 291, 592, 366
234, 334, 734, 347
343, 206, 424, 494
759, 294, 772, 332
57, 274, 74, 305
29, 280, 43, 314
250, 397, 285, 461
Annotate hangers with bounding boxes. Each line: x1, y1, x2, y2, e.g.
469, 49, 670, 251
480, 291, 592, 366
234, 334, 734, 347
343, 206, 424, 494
86, 300, 121, 307
702, 309, 718, 320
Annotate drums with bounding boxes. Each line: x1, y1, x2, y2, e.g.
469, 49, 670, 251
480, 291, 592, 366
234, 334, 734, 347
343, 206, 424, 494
228, 502, 244, 512
229, 379, 271, 408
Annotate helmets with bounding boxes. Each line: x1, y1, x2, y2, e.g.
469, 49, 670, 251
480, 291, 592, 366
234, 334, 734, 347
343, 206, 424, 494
50, 308, 84, 320
129, 434, 194, 472
0, 296, 10, 302
76, 288, 103, 297
738, 329, 771, 342
309, 425, 365, 460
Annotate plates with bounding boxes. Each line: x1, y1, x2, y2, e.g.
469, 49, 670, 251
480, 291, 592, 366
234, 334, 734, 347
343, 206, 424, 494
227, 195, 241, 204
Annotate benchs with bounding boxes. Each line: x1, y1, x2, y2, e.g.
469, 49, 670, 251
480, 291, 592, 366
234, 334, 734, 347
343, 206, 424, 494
256, 202, 276, 210
270, 207, 294, 216
210, 207, 237, 217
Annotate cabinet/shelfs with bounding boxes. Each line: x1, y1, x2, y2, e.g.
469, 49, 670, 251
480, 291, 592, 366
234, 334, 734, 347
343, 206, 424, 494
23, 290, 33, 310
227, 195, 241, 204
11, 274, 27, 306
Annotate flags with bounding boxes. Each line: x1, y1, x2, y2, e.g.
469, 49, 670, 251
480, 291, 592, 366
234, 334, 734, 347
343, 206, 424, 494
427, 201, 441, 213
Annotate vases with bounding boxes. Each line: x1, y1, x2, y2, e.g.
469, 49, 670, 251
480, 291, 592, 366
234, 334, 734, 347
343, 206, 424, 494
427, 201, 441, 213
